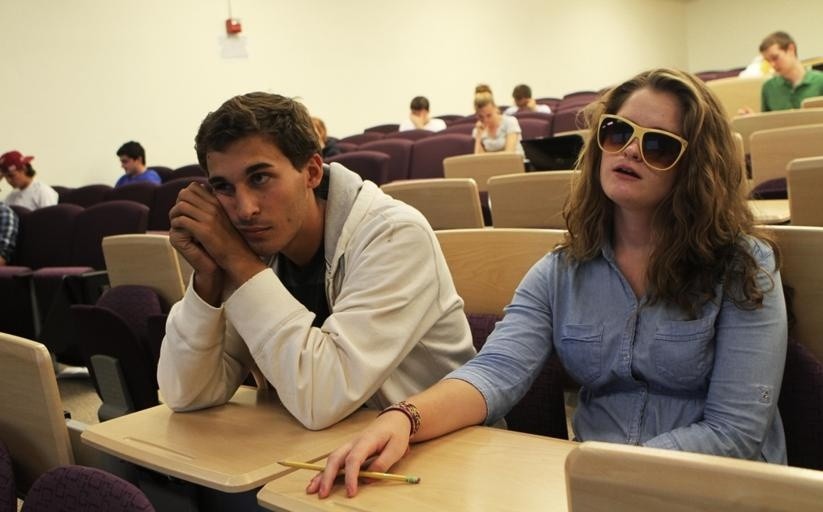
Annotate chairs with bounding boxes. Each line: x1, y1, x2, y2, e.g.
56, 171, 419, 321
496, 58, 823, 512
0, 115, 496, 512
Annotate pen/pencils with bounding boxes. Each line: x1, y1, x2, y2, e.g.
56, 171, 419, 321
276, 460, 420, 484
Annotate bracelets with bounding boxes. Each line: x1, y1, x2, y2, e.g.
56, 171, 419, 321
376, 401, 422, 437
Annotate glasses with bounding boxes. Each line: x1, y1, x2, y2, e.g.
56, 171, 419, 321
596, 111, 691, 173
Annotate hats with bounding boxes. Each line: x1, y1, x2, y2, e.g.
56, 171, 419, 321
0, 150, 36, 173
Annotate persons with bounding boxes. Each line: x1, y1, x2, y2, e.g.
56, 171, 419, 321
114, 141, 162, 189
0, 151, 59, 212
398, 83, 552, 162
311, 117, 339, 158
307, 68, 788, 469
0, 202, 19, 266
157, 91, 510, 432
737, 32, 823, 116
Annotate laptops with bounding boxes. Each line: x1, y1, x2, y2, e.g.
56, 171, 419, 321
520, 134, 586, 171
